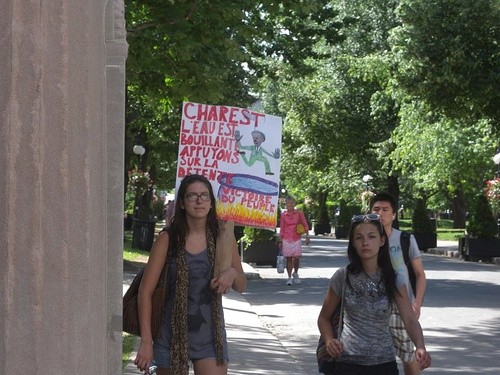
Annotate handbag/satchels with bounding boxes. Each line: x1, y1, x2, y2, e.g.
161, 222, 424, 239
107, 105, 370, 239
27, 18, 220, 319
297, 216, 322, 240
123, 268, 168, 340
315, 303, 345, 373
276, 250, 285, 274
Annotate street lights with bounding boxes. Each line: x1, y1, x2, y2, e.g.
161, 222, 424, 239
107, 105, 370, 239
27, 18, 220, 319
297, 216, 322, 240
132, 144, 146, 217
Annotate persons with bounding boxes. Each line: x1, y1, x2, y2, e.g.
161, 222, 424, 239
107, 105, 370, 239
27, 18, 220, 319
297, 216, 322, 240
134, 175, 247, 375
280, 196, 311, 285
371, 193, 426, 375
318, 215, 426, 375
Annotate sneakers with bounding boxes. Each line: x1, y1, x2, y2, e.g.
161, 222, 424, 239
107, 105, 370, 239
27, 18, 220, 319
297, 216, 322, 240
286, 277, 293, 286
292, 272, 301, 284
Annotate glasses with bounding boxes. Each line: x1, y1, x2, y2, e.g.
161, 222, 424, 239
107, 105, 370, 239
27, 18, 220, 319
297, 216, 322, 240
351, 213, 384, 235
186, 192, 210, 202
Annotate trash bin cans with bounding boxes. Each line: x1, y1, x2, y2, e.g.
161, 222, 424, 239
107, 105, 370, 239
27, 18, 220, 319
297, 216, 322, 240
131, 217, 156, 251
